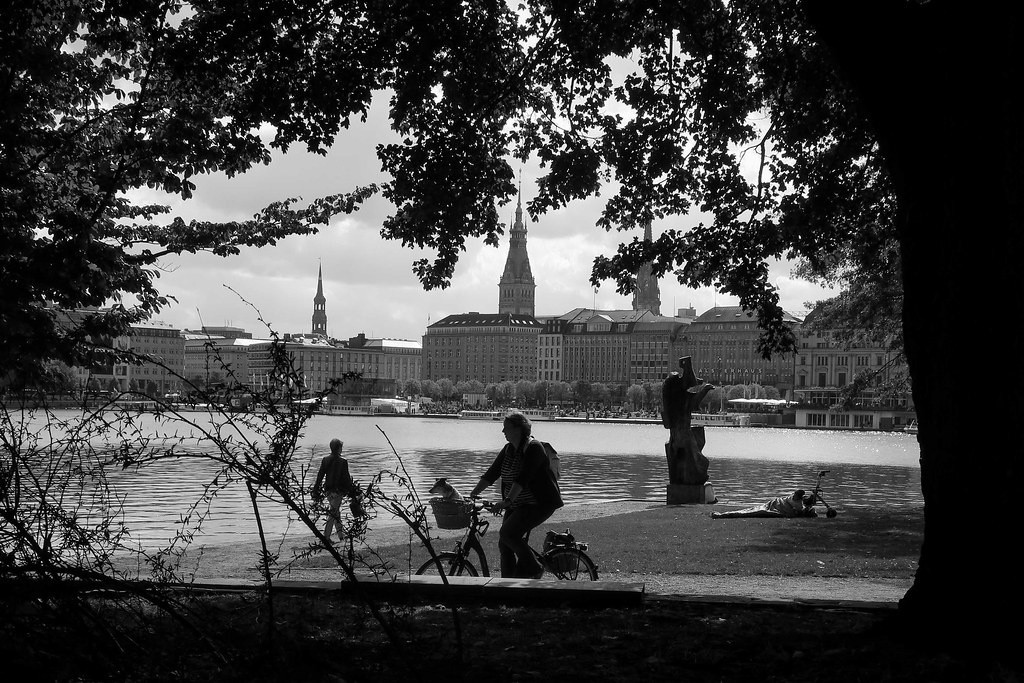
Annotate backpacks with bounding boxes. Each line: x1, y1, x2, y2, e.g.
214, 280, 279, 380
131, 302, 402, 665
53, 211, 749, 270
523, 436, 561, 482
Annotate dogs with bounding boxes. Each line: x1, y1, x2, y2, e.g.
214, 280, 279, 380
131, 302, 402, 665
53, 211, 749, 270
428, 478, 467, 515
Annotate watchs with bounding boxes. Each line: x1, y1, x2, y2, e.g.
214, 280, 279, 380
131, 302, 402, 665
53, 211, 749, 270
504, 497, 514, 505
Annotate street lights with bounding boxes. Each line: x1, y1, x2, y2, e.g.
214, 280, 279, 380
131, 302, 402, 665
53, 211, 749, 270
721, 385, 725, 412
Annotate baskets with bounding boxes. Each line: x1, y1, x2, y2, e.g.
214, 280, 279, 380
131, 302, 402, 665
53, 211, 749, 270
428, 496, 475, 529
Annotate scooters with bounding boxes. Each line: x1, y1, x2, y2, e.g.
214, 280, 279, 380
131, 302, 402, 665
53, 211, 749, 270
800, 469, 838, 518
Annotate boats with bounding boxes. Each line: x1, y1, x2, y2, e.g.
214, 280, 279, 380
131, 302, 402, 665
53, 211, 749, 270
506, 410, 557, 421
459, 410, 504, 420
904, 420, 920, 434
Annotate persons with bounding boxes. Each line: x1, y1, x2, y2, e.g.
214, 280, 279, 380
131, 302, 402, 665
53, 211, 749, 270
710, 490, 817, 518
314, 438, 352, 543
471, 412, 564, 578
419, 400, 808, 420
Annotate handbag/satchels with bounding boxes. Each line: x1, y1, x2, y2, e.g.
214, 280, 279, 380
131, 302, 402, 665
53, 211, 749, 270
541, 528, 577, 574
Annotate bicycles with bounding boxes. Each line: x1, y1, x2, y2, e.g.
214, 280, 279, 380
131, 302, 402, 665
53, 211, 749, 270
413, 496, 600, 582
